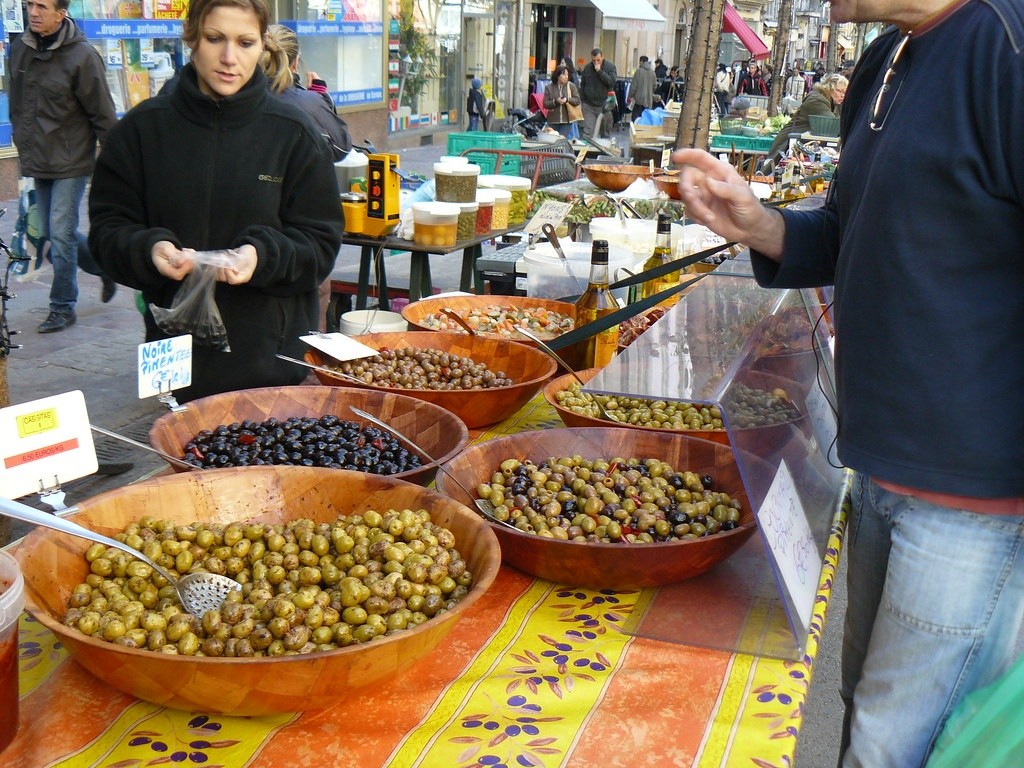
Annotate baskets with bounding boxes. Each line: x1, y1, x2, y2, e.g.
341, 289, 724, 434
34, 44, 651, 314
710, 136, 774, 151
520, 139, 576, 187
809, 115, 841, 137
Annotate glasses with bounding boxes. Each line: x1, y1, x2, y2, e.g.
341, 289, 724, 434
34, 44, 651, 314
867, 34, 911, 131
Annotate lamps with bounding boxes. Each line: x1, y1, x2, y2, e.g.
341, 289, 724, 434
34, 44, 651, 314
397, 52, 423, 111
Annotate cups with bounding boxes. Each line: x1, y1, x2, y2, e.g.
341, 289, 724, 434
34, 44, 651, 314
0, 549, 25, 754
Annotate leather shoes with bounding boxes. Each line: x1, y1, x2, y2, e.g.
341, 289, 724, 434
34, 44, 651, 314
39, 309, 76, 333
101, 272, 115, 303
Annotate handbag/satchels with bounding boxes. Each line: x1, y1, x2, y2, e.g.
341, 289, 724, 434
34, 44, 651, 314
565, 82, 584, 123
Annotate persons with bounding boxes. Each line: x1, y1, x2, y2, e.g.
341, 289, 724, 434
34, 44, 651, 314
9, 1, 118, 332
258, 25, 353, 332
759, 75, 849, 176
627, 56, 657, 124
660, 66, 684, 107
467, 79, 485, 131
580, 48, 617, 139
783, 62, 826, 103
672, 0, 1024, 768
837, 58, 854, 79
543, 67, 581, 137
655, 59, 668, 81
89, 0, 344, 405
561, 58, 580, 140
714, 63, 773, 118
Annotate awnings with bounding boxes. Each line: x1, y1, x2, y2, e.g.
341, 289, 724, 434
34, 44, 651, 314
837, 36, 853, 52
724, 2, 771, 60
590, 0, 666, 31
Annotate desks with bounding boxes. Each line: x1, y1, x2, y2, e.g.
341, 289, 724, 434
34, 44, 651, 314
655, 135, 675, 149
709, 147, 769, 155
473, 239, 526, 294
335, 220, 531, 312
331, 244, 497, 332
801, 130, 841, 150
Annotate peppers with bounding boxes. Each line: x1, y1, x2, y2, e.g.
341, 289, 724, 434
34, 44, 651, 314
528, 190, 615, 222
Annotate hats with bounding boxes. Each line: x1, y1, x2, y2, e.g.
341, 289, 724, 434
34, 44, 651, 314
472, 79, 482, 89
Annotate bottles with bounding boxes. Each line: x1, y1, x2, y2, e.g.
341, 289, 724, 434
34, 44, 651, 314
784, 166, 805, 204
768, 171, 785, 209
641, 213, 681, 307
805, 152, 825, 197
573, 239, 620, 371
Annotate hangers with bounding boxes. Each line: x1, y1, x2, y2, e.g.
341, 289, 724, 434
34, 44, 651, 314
614, 76, 632, 83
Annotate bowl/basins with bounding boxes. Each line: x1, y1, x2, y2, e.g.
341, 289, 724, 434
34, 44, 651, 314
15, 294, 839, 718
580, 163, 664, 191
653, 176, 683, 200
412, 155, 531, 247
536, 132, 560, 145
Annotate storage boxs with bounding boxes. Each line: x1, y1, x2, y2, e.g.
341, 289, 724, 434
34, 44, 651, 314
469, 158, 521, 176
663, 116, 680, 135
447, 130, 523, 157
752, 136, 776, 150
521, 138, 576, 187
710, 133, 751, 149
